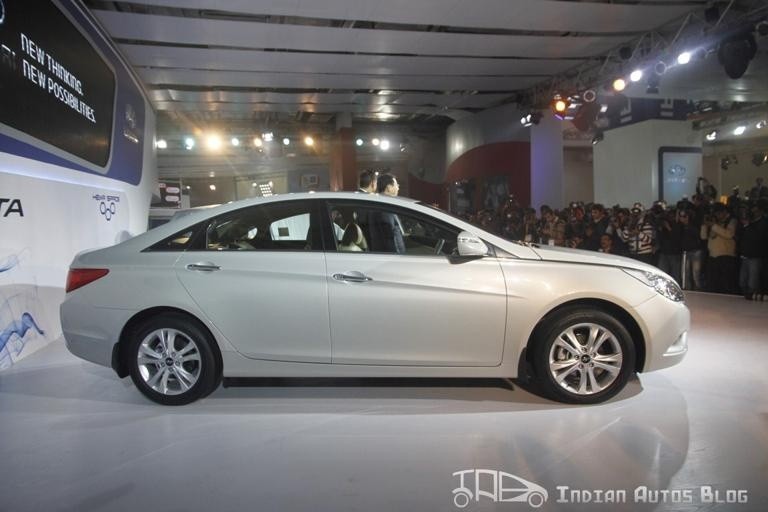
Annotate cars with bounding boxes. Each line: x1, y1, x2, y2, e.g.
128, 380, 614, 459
58, 189, 694, 405
145, 202, 188, 238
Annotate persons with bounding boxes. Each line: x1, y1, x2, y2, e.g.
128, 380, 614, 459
356, 171, 377, 251
368, 173, 406, 254
477, 177, 768, 301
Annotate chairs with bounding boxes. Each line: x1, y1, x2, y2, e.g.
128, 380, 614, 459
209, 221, 240, 250
338, 222, 364, 251
232, 224, 258, 250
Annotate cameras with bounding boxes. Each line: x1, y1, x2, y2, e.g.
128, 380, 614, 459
742, 210, 753, 220
706, 214, 719, 223
610, 215, 620, 224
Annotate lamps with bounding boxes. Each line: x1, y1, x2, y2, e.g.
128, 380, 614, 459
519, 45, 715, 128
